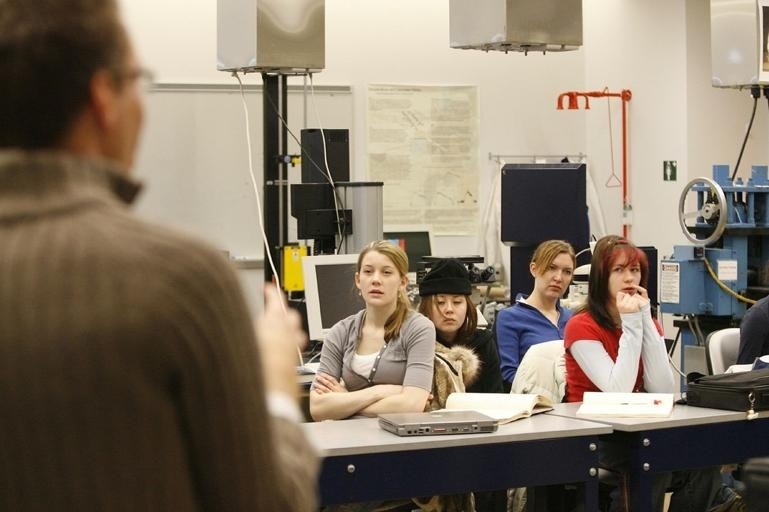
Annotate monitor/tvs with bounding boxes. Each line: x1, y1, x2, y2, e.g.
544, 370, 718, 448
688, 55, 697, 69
302, 254, 366, 342
386, 225, 436, 280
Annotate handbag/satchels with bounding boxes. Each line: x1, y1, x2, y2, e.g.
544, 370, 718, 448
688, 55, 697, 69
686, 367, 769, 412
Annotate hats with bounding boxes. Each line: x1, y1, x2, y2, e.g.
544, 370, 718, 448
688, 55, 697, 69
419, 258, 472, 296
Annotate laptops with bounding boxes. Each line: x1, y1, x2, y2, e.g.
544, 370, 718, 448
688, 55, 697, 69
378, 410, 500, 437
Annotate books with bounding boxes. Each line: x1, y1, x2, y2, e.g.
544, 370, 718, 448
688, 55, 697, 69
576, 383, 676, 421
433, 388, 555, 424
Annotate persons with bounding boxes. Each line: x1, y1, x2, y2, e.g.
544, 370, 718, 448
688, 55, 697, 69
493, 238, 588, 511
306, 238, 434, 512
416, 256, 507, 511
2, 1, 321, 510
563, 235, 724, 512
708, 290, 769, 511
